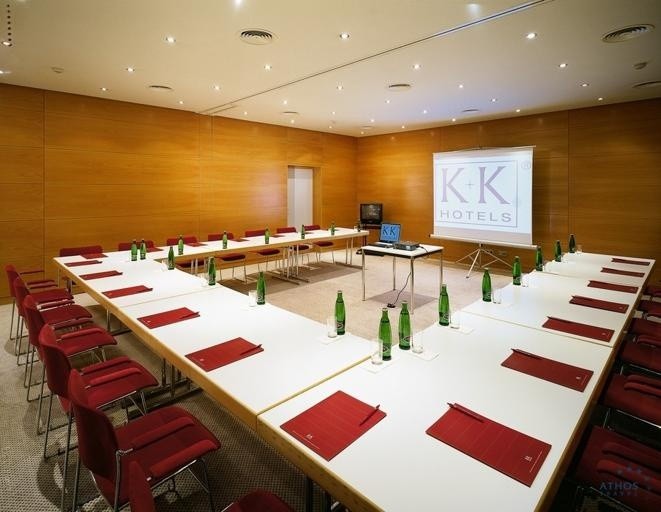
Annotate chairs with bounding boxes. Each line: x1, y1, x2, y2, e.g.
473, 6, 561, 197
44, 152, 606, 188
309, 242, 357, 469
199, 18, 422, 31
552, 272, 661, 510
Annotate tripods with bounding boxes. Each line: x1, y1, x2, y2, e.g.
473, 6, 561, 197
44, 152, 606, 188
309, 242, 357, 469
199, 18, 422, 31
455, 243, 513, 279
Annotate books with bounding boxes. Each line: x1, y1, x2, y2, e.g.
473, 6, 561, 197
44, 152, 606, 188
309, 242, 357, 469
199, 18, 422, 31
63, 260, 100, 267
501, 349, 595, 394
78, 270, 123, 280
568, 295, 629, 313
611, 257, 651, 267
426, 402, 552, 488
542, 315, 615, 342
80, 229, 342, 260
280, 390, 387, 461
599, 267, 645, 278
185, 337, 264, 374
101, 285, 153, 299
138, 306, 201, 330
587, 281, 638, 294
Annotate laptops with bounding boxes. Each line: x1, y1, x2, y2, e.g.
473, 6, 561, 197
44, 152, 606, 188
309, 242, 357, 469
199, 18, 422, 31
368, 223, 401, 248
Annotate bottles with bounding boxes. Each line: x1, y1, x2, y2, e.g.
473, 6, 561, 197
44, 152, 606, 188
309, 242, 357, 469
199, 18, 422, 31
413, 234, 584, 356
397, 301, 412, 351
219, 218, 361, 249
378, 308, 393, 360
127, 234, 216, 287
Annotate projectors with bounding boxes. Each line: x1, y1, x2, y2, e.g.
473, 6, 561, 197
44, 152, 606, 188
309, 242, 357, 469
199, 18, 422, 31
394, 240, 420, 251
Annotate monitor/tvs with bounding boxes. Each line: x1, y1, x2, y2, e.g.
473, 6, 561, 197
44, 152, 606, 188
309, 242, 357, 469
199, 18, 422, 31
360, 204, 382, 224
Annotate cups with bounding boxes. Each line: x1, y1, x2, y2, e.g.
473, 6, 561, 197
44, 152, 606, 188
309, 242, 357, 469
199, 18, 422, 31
159, 260, 345, 340
369, 339, 384, 364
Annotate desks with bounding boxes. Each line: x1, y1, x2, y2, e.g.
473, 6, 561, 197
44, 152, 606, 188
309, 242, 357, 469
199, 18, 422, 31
356, 237, 443, 314
